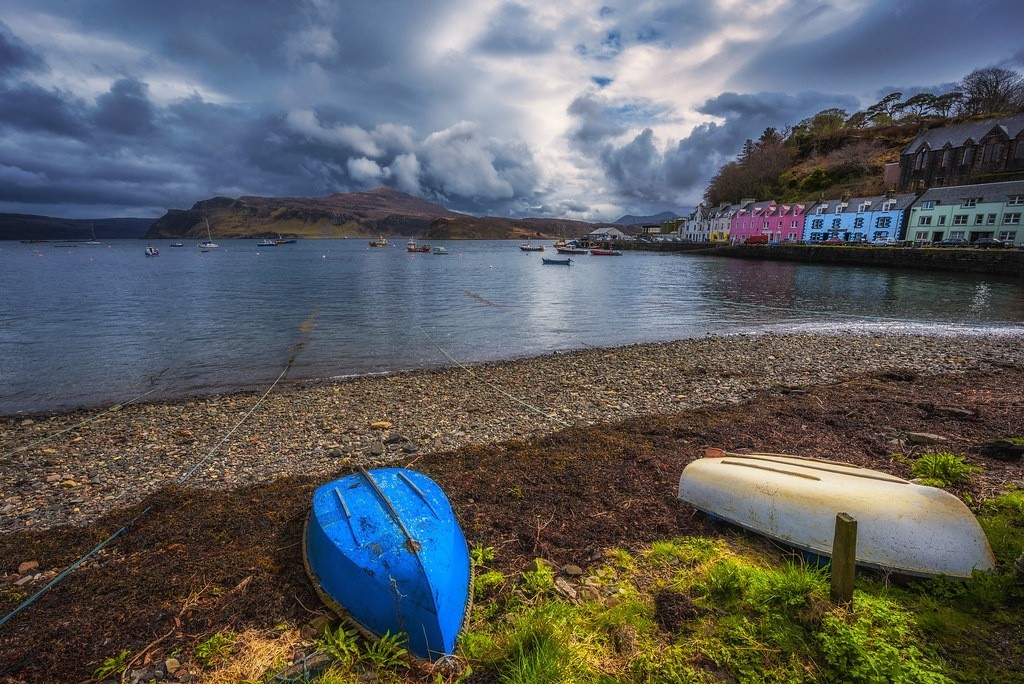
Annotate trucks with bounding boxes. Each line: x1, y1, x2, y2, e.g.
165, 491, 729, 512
743, 235, 769, 245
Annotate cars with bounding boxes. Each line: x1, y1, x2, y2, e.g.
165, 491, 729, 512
579, 236, 682, 242
818, 237, 847, 246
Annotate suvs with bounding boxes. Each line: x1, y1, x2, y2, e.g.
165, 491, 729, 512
870, 237, 897, 248
972, 238, 1003, 249
933, 237, 969, 248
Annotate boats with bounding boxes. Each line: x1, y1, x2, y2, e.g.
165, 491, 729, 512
557, 248, 589, 255
303, 467, 475, 676
590, 249, 623, 256
541, 257, 574, 266
275, 239, 297, 244
407, 237, 432, 252
677, 445, 998, 586
553, 238, 567, 248
258, 239, 278, 246
145, 246, 160, 257
170, 242, 183, 248
197, 218, 219, 252
368, 235, 390, 248
433, 246, 448, 254
518, 242, 544, 251
